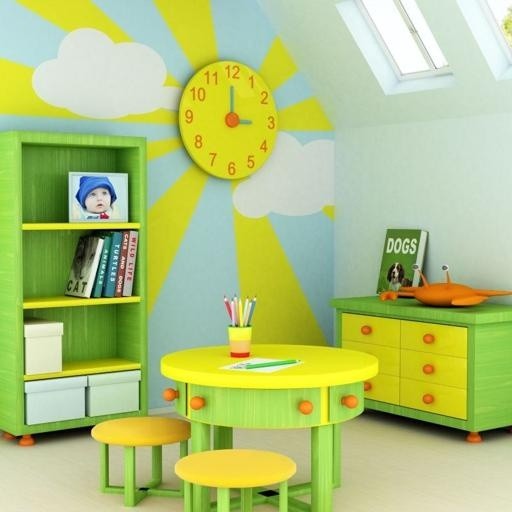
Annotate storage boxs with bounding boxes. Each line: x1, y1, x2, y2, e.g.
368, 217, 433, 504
26, 370, 143, 425
22, 319, 64, 375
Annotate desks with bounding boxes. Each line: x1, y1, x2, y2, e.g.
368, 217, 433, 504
157, 342, 380, 512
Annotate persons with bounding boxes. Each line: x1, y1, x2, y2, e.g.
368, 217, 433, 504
74, 177, 118, 221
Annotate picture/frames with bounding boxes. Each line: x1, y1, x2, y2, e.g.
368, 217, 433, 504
65, 170, 131, 224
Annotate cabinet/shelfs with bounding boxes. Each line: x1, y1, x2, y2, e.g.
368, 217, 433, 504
0, 130, 147, 446
336, 307, 511, 444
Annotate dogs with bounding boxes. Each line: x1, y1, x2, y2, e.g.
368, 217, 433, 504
386, 262, 412, 293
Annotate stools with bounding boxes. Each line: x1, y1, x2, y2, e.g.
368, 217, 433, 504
91, 416, 298, 511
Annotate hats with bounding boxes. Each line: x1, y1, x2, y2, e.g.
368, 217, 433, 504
76, 176, 116, 208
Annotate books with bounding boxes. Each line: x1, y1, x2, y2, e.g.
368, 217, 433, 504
374, 227, 430, 300
64, 230, 140, 299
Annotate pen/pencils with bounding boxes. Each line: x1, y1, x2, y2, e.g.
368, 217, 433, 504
246, 360, 301, 368
225, 294, 257, 327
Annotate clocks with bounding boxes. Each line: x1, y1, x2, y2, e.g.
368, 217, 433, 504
175, 59, 280, 180
